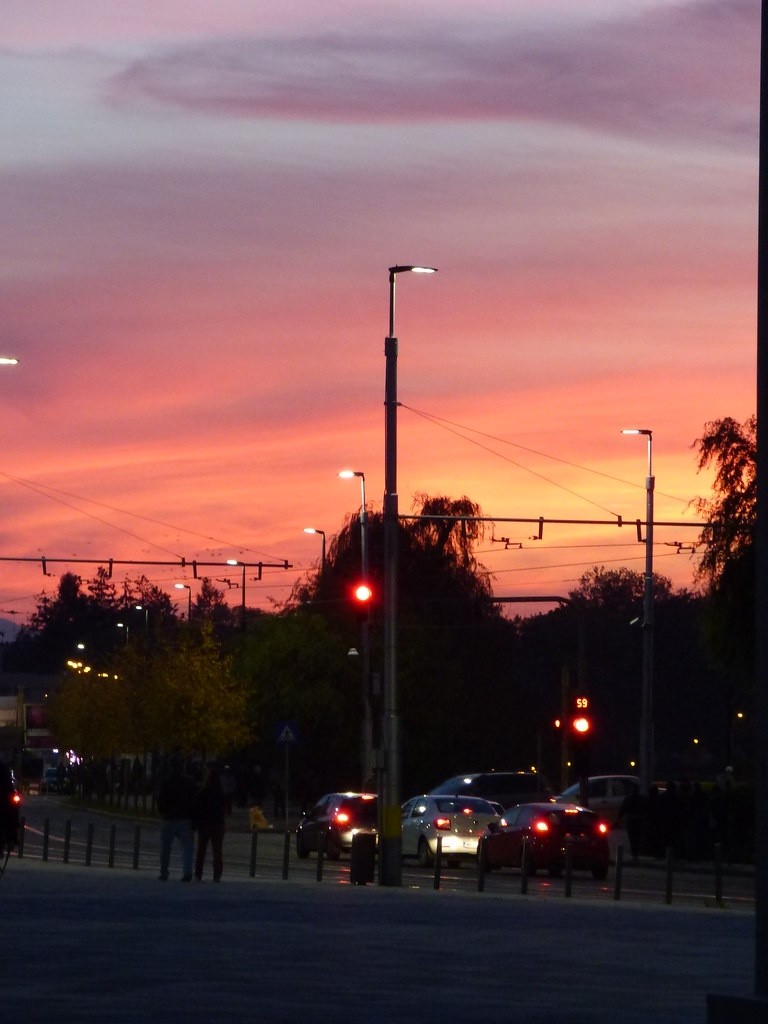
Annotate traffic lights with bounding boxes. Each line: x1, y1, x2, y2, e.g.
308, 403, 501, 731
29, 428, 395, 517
575, 693, 591, 733
355, 586, 372, 604
552, 718, 562, 732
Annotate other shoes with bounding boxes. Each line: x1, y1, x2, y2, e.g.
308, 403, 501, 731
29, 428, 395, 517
180, 875, 192, 882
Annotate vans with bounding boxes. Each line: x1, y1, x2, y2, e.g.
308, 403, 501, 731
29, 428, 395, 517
427, 771, 552, 817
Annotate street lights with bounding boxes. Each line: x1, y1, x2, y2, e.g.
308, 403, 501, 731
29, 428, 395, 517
174, 582, 191, 621
135, 604, 150, 639
339, 471, 374, 794
302, 526, 329, 600
117, 622, 130, 649
621, 429, 656, 779
375, 263, 442, 890
225, 558, 249, 630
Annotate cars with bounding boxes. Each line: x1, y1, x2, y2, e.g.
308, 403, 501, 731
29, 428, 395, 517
474, 802, 613, 877
399, 796, 501, 869
38, 766, 71, 793
483, 798, 507, 820
295, 791, 381, 860
0, 761, 26, 874
551, 773, 651, 830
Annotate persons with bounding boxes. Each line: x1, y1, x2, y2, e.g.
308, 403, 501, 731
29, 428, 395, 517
150, 764, 287, 883
616, 776, 733, 860
